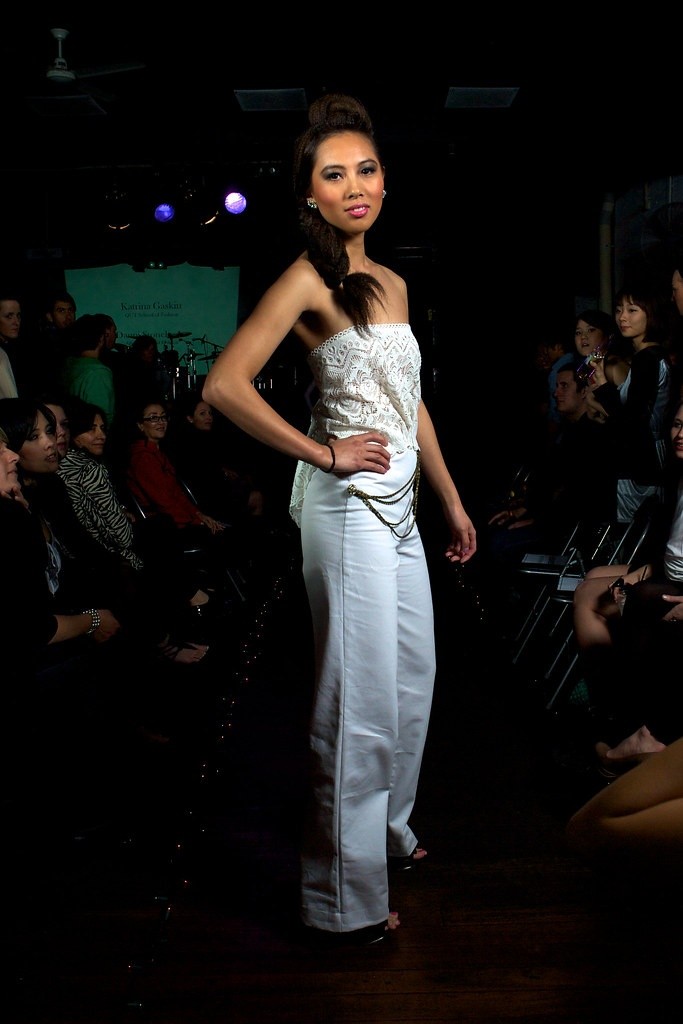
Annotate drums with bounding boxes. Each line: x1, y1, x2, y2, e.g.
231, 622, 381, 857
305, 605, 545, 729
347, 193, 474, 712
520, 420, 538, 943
160, 350, 180, 369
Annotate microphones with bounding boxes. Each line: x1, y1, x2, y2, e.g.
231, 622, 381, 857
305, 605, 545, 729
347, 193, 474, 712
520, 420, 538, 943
201, 335, 205, 343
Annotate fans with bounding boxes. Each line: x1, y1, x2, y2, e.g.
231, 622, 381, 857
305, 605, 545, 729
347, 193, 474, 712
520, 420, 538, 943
0, 28, 147, 114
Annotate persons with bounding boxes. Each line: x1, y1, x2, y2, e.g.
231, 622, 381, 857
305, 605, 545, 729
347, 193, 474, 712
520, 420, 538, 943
0, 281, 267, 747
453, 265, 683, 863
196, 94, 480, 951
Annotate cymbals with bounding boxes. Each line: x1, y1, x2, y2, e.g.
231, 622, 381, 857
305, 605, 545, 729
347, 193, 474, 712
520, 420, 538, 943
198, 355, 218, 361
168, 331, 193, 339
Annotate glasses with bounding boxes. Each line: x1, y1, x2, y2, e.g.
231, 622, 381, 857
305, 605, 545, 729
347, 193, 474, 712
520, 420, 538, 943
143, 415, 168, 422
613, 578, 631, 595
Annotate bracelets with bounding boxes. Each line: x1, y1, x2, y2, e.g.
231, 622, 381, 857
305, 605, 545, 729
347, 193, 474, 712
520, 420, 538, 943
319, 442, 336, 473
81, 608, 100, 636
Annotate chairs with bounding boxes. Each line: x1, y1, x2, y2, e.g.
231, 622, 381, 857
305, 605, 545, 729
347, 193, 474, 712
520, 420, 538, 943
129, 481, 245, 616
504, 494, 662, 731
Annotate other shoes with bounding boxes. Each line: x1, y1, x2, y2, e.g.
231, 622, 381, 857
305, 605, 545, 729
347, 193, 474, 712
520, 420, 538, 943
192, 586, 218, 617
363, 911, 401, 934
388, 844, 428, 867
156, 639, 209, 668
594, 741, 657, 778
